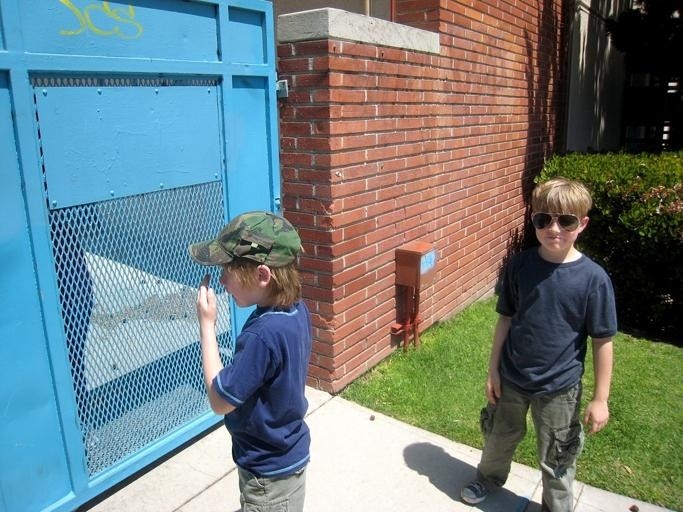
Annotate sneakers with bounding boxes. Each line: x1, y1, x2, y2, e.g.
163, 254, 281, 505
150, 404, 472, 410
461, 480, 488, 504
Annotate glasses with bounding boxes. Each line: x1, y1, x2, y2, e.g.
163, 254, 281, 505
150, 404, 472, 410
531, 212, 582, 232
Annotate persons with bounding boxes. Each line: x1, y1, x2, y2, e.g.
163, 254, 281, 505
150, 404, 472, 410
187, 211, 313, 511
51, 205, 106, 453
458, 176, 618, 511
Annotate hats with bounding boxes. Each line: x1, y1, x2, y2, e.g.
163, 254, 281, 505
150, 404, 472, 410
187, 211, 301, 268
